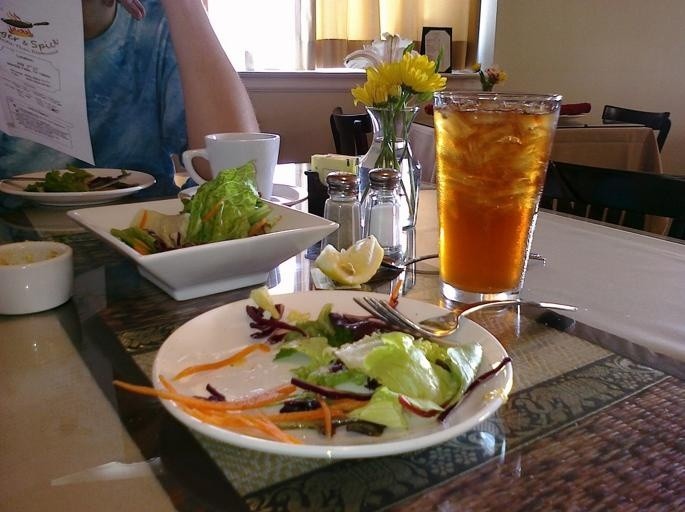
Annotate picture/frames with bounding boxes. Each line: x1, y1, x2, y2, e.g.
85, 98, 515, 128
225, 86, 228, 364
421, 26, 453, 73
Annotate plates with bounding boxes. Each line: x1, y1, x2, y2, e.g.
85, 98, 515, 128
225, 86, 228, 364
148, 290, 515, 459
0, 165, 163, 206
174, 178, 307, 210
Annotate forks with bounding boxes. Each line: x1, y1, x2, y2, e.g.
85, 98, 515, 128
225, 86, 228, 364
371, 249, 548, 274
351, 289, 577, 334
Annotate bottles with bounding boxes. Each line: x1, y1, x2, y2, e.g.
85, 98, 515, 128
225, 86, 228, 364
317, 166, 402, 263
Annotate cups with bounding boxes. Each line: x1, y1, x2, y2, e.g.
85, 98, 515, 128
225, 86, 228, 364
304, 171, 330, 218
181, 128, 280, 202
0, 240, 71, 319
432, 86, 565, 303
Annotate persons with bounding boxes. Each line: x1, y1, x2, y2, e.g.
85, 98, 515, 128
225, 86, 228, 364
0, 1, 262, 211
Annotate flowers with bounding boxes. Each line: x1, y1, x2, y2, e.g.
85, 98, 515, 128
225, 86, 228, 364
471, 62, 509, 92
344, 30, 450, 217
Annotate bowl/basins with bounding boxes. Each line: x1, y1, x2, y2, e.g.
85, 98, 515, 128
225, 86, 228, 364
63, 191, 340, 302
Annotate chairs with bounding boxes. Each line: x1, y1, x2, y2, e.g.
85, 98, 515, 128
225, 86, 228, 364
328, 105, 374, 155
603, 105, 673, 154
557, 102, 592, 116
538, 160, 685, 243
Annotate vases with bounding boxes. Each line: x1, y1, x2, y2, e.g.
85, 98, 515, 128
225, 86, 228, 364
356, 101, 424, 227
